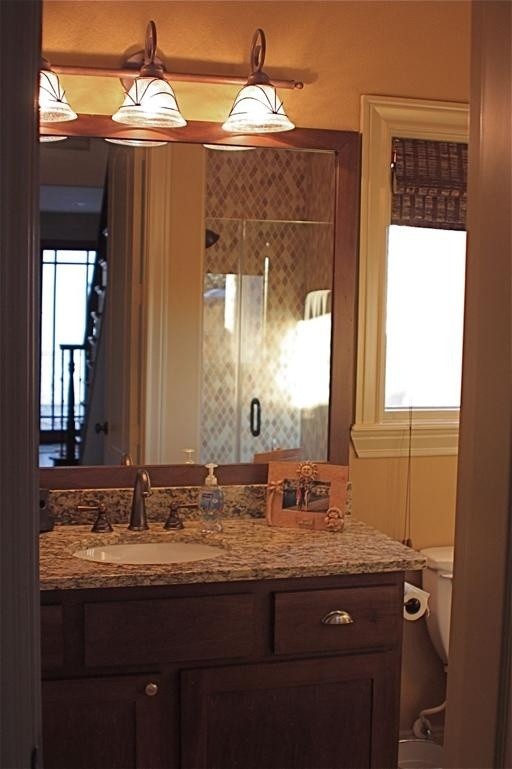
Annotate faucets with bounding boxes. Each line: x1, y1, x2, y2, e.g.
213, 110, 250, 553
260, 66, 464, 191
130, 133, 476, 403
128, 468, 153, 531
119, 452, 134, 467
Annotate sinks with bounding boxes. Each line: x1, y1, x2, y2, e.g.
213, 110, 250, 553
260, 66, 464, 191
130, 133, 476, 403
65, 532, 232, 566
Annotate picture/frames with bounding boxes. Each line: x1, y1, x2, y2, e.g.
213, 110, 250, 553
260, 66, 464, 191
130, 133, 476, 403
264, 459, 349, 534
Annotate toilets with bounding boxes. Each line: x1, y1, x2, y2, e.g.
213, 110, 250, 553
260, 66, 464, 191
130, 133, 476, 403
418, 544, 455, 673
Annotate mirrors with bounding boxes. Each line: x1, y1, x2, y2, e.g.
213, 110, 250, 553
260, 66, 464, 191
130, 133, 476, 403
35, 114, 362, 491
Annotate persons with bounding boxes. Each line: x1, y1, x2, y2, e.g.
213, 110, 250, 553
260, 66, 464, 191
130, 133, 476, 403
296, 482, 305, 510
302, 485, 310, 509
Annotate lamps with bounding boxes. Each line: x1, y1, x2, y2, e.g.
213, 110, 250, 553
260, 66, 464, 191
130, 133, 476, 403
36, 18, 306, 138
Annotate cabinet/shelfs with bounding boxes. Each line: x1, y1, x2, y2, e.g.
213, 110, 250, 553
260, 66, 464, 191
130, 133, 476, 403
41, 572, 406, 769
